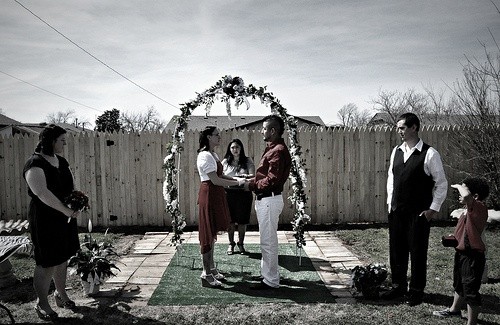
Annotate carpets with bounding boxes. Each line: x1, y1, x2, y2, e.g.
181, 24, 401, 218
147, 242, 339, 306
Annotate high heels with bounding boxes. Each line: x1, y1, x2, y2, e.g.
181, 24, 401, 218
210, 268, 225, 279
54, 289, 75, 308
227, 242, 236, 255
200, 272, 222, 287
237, 242, 246, 254
35, 304, 58, 319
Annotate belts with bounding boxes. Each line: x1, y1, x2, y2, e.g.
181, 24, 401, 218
255, 190, 281, 200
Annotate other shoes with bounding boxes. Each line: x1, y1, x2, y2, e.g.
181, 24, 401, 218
406, 289, 424, 301
442, 309, 462, 317
252, 275, 263, 281
253, 281, 276, 291
381, 283, 407, 300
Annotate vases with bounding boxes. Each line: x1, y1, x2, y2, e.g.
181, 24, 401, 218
81, 270, 99, 296
362, 284, 380, 300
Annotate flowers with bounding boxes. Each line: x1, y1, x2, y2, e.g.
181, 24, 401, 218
162, 74, 312, 266
63, 190, 91, 222
349, 263, 399, 299
67, 219, 127, 281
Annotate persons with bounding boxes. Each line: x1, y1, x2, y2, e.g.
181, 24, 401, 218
245, 115, 292, 289
23, 124, 88, 320
220, 138, 255, 255
195, 125, 250, 287
433, 177, 490, 325
386, 113, 448, 305
